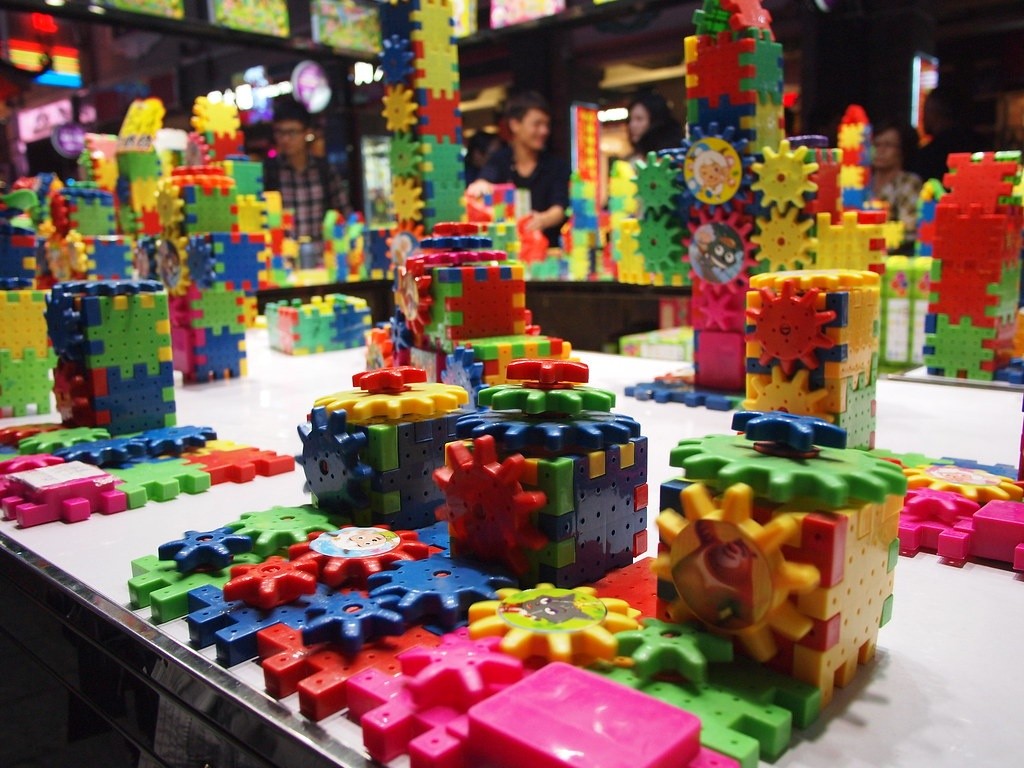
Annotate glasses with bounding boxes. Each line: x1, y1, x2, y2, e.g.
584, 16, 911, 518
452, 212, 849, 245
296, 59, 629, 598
274, 129, 305, 140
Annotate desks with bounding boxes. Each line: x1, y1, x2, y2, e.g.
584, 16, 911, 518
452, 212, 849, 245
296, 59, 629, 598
0, 332, 1024, 768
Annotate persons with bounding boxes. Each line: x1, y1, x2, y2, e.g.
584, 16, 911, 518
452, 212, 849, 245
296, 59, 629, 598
264, 102, 354, 266
627, 94, 684, 164
464, 89, 568, 247
871, 114, 923, 257
